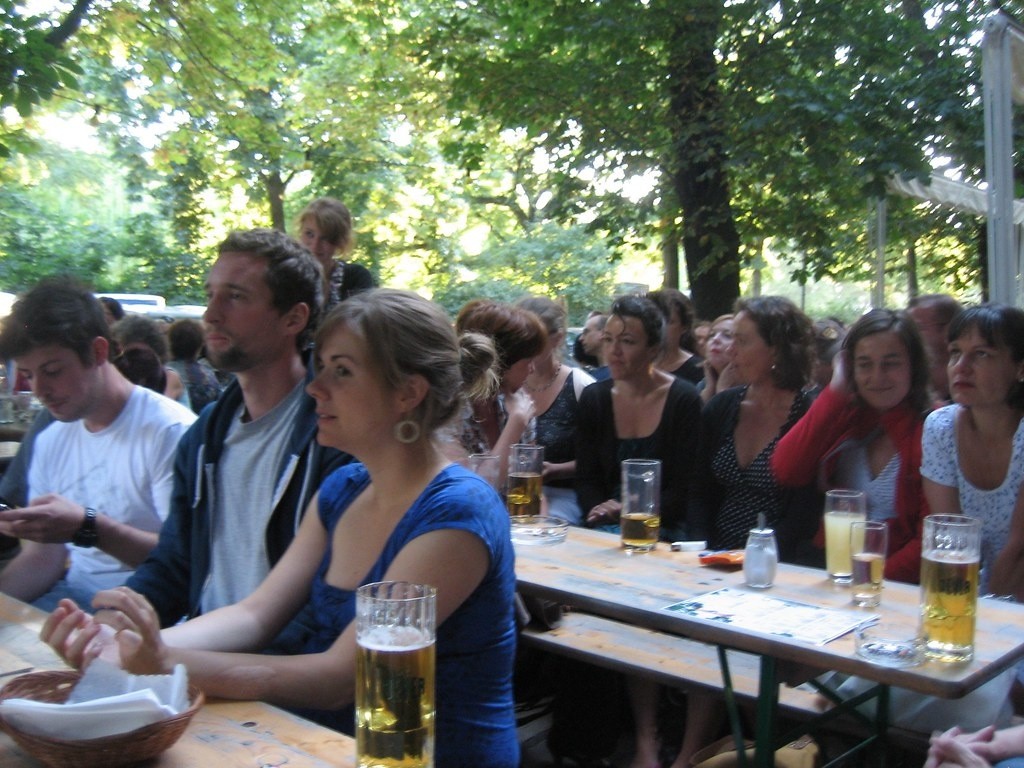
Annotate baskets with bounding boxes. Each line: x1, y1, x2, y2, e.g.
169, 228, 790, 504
0, 671, 204, 768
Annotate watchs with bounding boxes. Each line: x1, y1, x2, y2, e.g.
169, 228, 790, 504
71, 507, 99, 548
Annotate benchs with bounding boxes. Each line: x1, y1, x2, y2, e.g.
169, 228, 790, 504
528, 616, 879, 763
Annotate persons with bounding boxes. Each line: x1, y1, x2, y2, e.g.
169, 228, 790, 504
0, 229, 1024, 768
296, 199, 377, 368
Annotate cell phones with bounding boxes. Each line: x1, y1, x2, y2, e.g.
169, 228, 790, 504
0, 504, 11, 512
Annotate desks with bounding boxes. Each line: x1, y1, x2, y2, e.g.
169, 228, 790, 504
508, 529, 1024, 768
0, 593, 360, 767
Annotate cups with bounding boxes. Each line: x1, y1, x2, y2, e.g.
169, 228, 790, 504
468, 453, 501, 495
823, 489, 866, 585
849, 520, 888, 609
921, 512, 982, 664
352, 581, 438, 768
619, 458, 662, 554
505, 443, 544, 524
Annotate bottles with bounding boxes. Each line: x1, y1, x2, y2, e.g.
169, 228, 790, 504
742, 526, 778, 591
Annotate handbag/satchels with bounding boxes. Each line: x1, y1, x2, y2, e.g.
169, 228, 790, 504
690, 732, 822, 768
